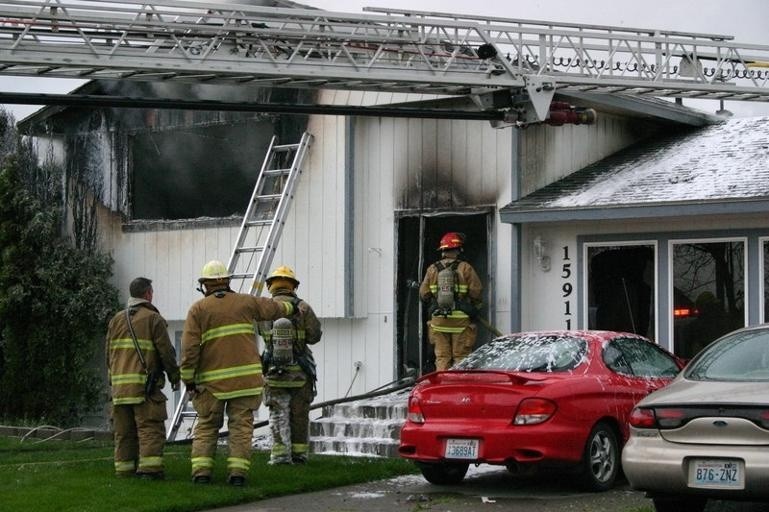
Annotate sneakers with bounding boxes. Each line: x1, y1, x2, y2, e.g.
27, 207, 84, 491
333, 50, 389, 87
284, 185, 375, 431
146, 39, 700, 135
115, 469, 164, 479
229, 476, 245, 486
267, 456, 306, 464
195, 476, 210, 483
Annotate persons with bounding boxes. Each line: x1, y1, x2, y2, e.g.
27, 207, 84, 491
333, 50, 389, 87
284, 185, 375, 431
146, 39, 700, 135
253, 266, 324, 465
103, 276, 183, 483
418, 233, 485, 372
178, 259, 302, 484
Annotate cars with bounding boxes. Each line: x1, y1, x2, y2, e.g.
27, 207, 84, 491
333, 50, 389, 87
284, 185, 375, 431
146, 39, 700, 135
617, 321, 769, 511
396, 329, 685, 492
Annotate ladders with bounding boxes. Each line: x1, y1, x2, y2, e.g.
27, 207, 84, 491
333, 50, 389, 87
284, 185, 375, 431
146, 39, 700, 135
164, 133, 312, 447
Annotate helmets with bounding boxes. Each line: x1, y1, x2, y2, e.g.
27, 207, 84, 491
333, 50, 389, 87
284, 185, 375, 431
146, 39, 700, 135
198, 261, 233, 283
265, 266, 300, 285
436, 232, 464, 251
